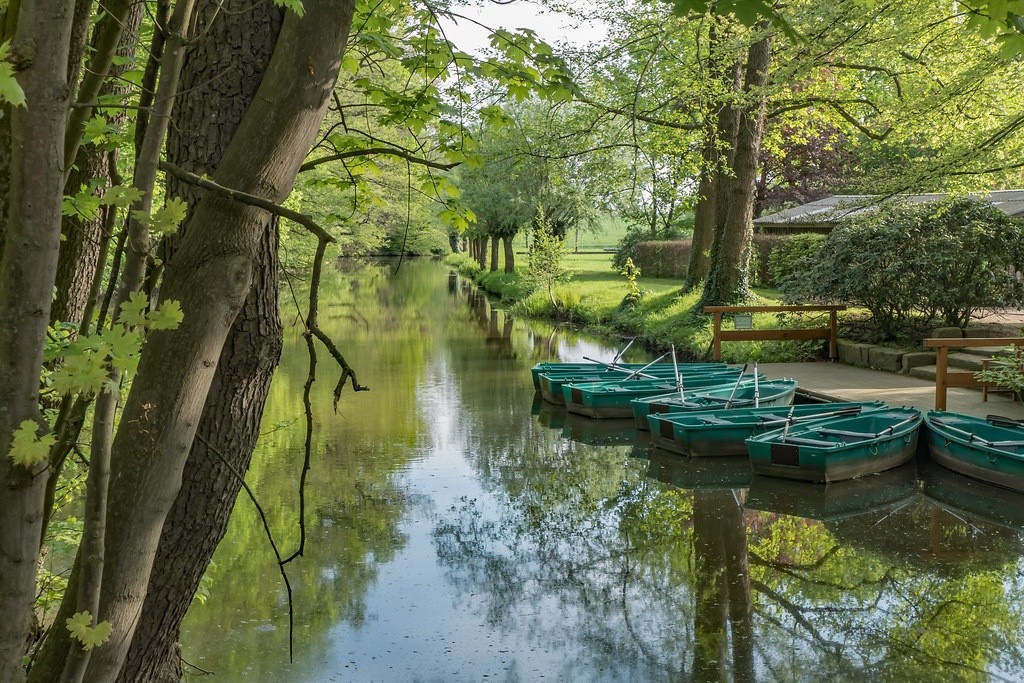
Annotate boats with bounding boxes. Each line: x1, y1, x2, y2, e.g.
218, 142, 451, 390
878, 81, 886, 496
530, 357, 764, 419
529, 390, 755, 490
630, 377, 796, 433
743, 404, 924, 485
917, 450, 1024, 534
925, 407, 1024, 503
646, 397, 885, 459
737, 443, 928, 522
